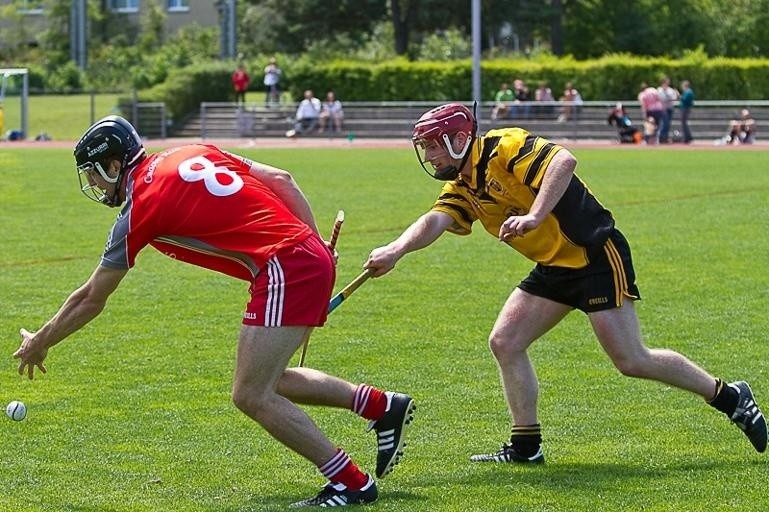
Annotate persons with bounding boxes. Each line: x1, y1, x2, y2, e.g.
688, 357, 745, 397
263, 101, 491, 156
493, 73, 695, 147
10, 111, 417, 510
231, 64, 249, 111
361, 100, 769, 472
723, 108, 755, 146
285, 89, 321, 135
317, 92, 345, 135
262, 58, 284, 109
6, 129, 25, 141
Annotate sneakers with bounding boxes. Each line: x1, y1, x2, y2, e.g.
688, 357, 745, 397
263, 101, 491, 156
367, 391, 416, 479
470, 443, 544, 465
288, 471, 377, 508
727, 381, 769, 452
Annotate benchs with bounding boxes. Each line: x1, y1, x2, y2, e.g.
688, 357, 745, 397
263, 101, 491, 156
174, 99, 769, 140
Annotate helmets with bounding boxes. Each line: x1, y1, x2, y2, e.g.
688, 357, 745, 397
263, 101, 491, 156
412, 104, 477, 180
74, 115, 147, 208
